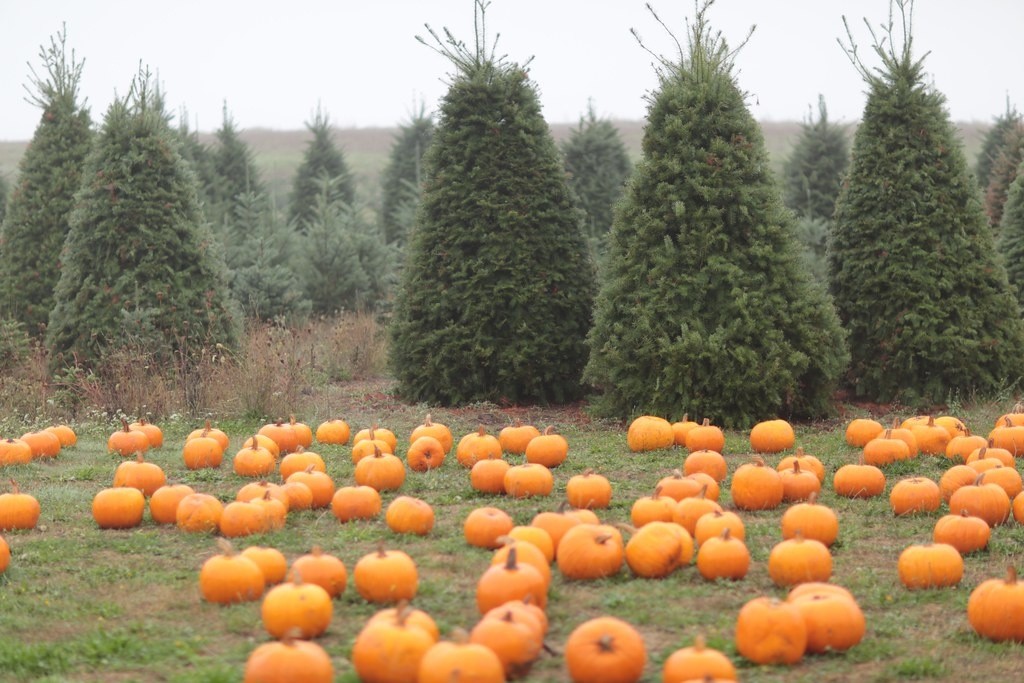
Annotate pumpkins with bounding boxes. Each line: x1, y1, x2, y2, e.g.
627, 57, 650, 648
0, 412, 1024, 683
